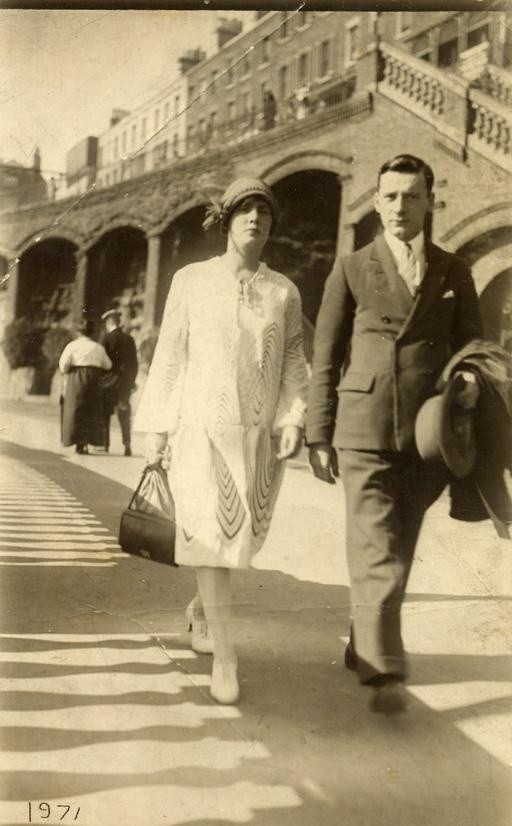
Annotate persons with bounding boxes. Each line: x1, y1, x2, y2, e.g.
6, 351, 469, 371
121, 175, 311, 707
301, 153, 484, 717
58, 319, 114, 456
96, 307, 140, 455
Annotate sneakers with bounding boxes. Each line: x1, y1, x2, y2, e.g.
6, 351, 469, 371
76, 441, 131, 456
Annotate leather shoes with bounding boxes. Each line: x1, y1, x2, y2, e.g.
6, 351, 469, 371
368, 680, 410, 719
344, 642, 385, 686
209, 650, 241, 704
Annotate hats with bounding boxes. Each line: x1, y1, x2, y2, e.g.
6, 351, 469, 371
100, 309, 121, 320
218, 177, 280, 233
415, 372, 481, 479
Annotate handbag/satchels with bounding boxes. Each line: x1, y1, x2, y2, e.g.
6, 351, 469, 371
99, 369, 126, 405
119, 462, 184, 569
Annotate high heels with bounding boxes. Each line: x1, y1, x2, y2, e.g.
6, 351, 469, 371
184, 601, 213, 655
